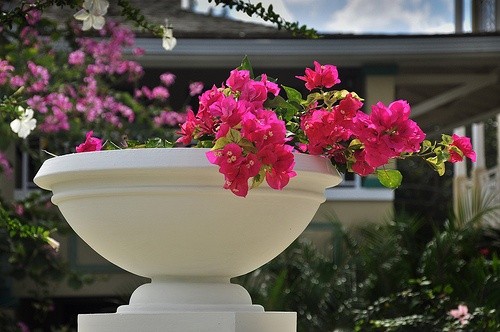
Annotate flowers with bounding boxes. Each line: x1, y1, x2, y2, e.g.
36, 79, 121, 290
40, 55, 476, 198
